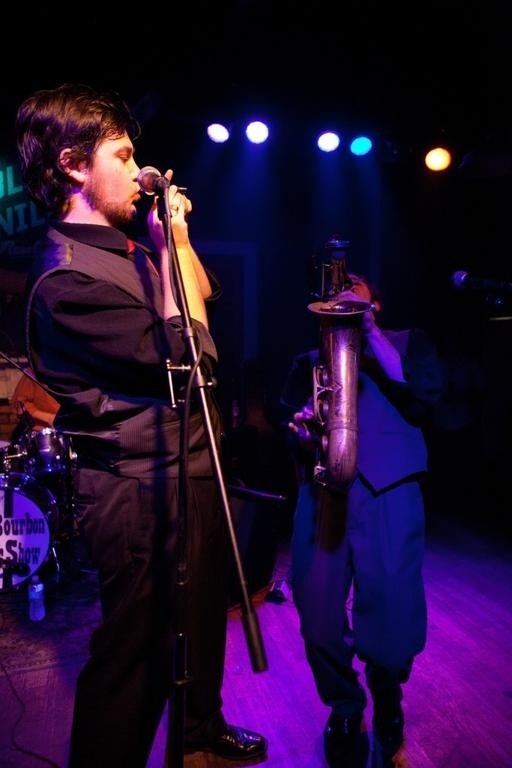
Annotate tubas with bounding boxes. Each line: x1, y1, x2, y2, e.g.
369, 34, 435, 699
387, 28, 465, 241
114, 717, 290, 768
306, 239, 375, 495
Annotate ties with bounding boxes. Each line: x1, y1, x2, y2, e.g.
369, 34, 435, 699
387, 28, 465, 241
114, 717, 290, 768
127, 238, 156, 306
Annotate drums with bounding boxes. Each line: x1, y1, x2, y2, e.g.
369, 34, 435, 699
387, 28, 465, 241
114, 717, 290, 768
0, 472, 59, 588
1, 441, 37, 487
27, 428, 71, 474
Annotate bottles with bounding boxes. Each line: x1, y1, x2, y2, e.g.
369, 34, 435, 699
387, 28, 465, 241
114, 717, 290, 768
28, 574, 47, 621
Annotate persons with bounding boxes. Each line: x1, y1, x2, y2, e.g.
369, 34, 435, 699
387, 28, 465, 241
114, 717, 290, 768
273, 261, 451, 768
8, 360, 93, 578
10, 81, 271, 768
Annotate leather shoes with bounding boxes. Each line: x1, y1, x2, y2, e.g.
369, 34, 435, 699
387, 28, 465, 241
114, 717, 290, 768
323, 687, 406, 768
183, 720, 268, 761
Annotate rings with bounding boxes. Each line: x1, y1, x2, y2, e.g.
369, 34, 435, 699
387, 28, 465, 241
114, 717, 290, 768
170, 204, 179, 211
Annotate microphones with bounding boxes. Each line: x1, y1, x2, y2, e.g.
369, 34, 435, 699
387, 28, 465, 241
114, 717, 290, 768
449, 269, 500, 305
137, 165, 188, 195
